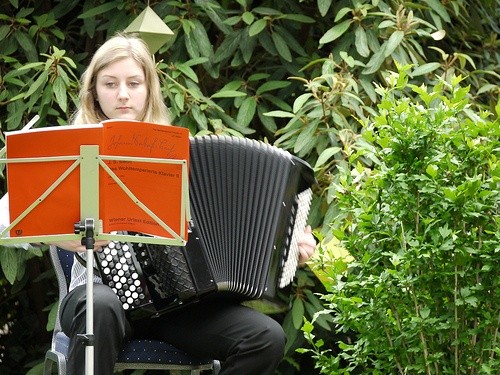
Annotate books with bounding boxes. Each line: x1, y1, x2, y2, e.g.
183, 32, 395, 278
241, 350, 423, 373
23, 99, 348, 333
5, 118, 190, 243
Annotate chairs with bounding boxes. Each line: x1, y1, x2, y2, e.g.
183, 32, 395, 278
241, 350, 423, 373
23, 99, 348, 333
43, 245, 221, 375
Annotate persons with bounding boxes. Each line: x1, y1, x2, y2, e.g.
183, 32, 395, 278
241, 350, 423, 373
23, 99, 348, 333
0, 35, 319, 375
